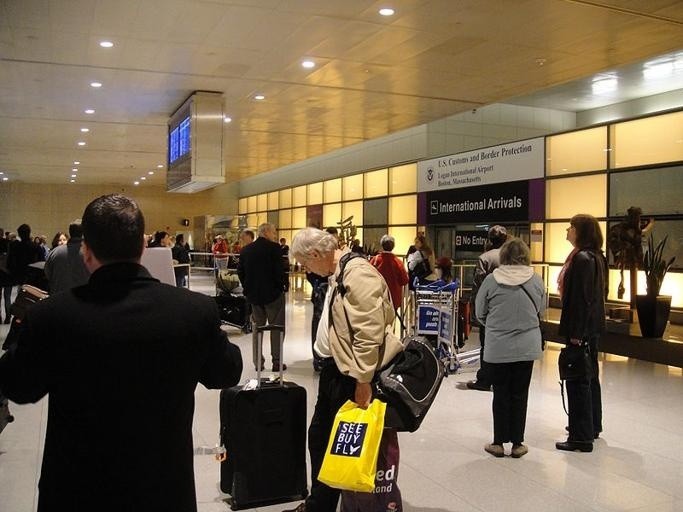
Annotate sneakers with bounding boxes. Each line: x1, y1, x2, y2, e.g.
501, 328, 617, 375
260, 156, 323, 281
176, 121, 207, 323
483, 442, 504, 458
510, 444, 529, 458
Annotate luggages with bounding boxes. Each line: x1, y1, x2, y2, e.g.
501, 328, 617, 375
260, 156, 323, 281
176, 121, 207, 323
218, 324, 308, 506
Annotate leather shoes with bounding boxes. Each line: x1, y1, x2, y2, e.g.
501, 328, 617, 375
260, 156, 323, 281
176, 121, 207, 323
272, 364, 287, 371
254, 362, 264, 372
467, 379, 492, 391
556, 440, 593, 453
564, 426, 599, 439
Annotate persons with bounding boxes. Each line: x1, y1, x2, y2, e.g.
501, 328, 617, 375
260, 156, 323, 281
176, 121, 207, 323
43, 221, 90, 293
351, 239, 362, 253
553, 213, 609, 453
406, 235, 455, 350
0, 192, 243, 510
473, 237, 548, 459
1, 223, 70, 325
281, 238, 289, 259
307, 228, 341, 373
48, 230, 69, 249
281, 226, 405, 511
237, 222, 290, 372
465, 225, 508, 392
148, 232, 191, 288
201, 229, 254, 277
370, 234, 410, 310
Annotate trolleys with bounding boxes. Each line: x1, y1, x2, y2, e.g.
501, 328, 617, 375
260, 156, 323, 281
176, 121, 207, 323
212, 251, 231, 277
411, 275, 463, 375
208, 266, 253, 335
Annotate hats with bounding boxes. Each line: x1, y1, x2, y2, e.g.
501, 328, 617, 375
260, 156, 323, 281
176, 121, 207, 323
435, 256, 452, 266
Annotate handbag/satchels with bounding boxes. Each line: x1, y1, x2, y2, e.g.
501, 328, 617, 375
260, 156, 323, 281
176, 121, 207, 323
339, 427, 404, 512
539, 324, 546, 352
559, 346, 595, 418
370, 335, 447, 436
316, 397, 388, 492
408, 250, 432, 291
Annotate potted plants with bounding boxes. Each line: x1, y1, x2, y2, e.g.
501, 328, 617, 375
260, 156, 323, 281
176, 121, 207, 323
625, 227, 677, 342
